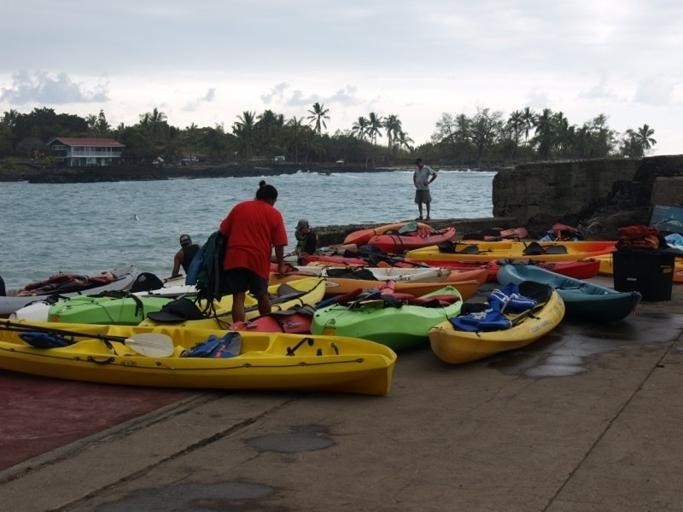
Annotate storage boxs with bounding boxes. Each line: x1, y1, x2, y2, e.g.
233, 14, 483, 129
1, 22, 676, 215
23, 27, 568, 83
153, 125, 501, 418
610, 247, 676, 305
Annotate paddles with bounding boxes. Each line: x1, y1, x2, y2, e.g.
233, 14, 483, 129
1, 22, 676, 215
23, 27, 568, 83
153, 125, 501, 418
2, 321, 173, 358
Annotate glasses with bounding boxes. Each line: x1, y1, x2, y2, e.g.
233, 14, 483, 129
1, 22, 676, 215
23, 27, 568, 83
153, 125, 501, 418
181, 243, 189, 246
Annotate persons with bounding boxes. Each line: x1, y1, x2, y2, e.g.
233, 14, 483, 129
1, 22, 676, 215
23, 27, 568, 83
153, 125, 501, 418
217, 180, 288, 325
411, 157, 436, 221
288, 217, 317, 265
168, 233, 201, 275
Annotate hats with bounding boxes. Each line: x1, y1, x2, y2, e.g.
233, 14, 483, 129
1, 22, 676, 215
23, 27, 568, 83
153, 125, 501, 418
295, 221, 308, 229
180, 234, 191, 245
